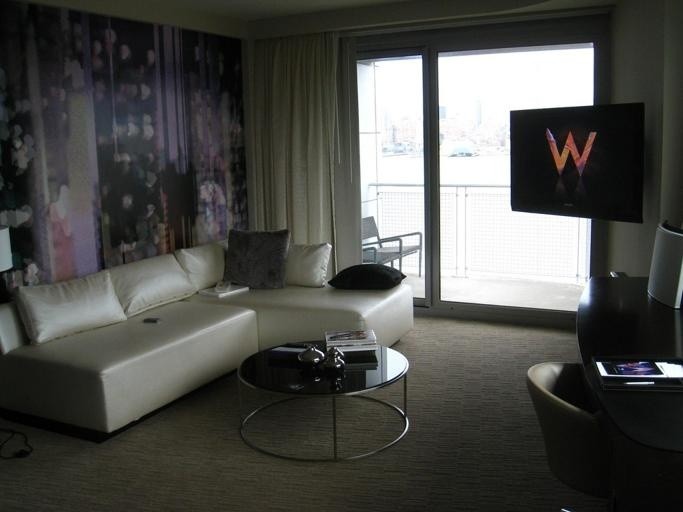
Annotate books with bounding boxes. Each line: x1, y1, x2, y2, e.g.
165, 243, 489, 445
324, 328, 379, 353
341, 351, 378, 371
199, 284, 249, 298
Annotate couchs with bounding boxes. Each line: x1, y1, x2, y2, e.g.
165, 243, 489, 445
196, 275, 413, 345
1, 282, 258, 439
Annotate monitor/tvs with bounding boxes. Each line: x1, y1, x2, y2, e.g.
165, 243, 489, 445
510, 101, 645, 225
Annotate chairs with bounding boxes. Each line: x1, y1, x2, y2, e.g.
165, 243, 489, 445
362, 216, 422, 277
526, 361, 659, 512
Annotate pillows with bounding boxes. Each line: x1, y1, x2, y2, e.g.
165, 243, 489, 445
327, 263, 405, 290
13, 269, 128, 345
109, 253, 198, 318
222, 229, 291, 288
174, 241, 226, 292
285, 242, 333, 287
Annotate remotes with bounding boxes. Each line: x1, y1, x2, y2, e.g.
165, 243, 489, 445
609, 271, 620, 278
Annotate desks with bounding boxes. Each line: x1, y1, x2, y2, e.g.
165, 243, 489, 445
574, 275, 683, 453
